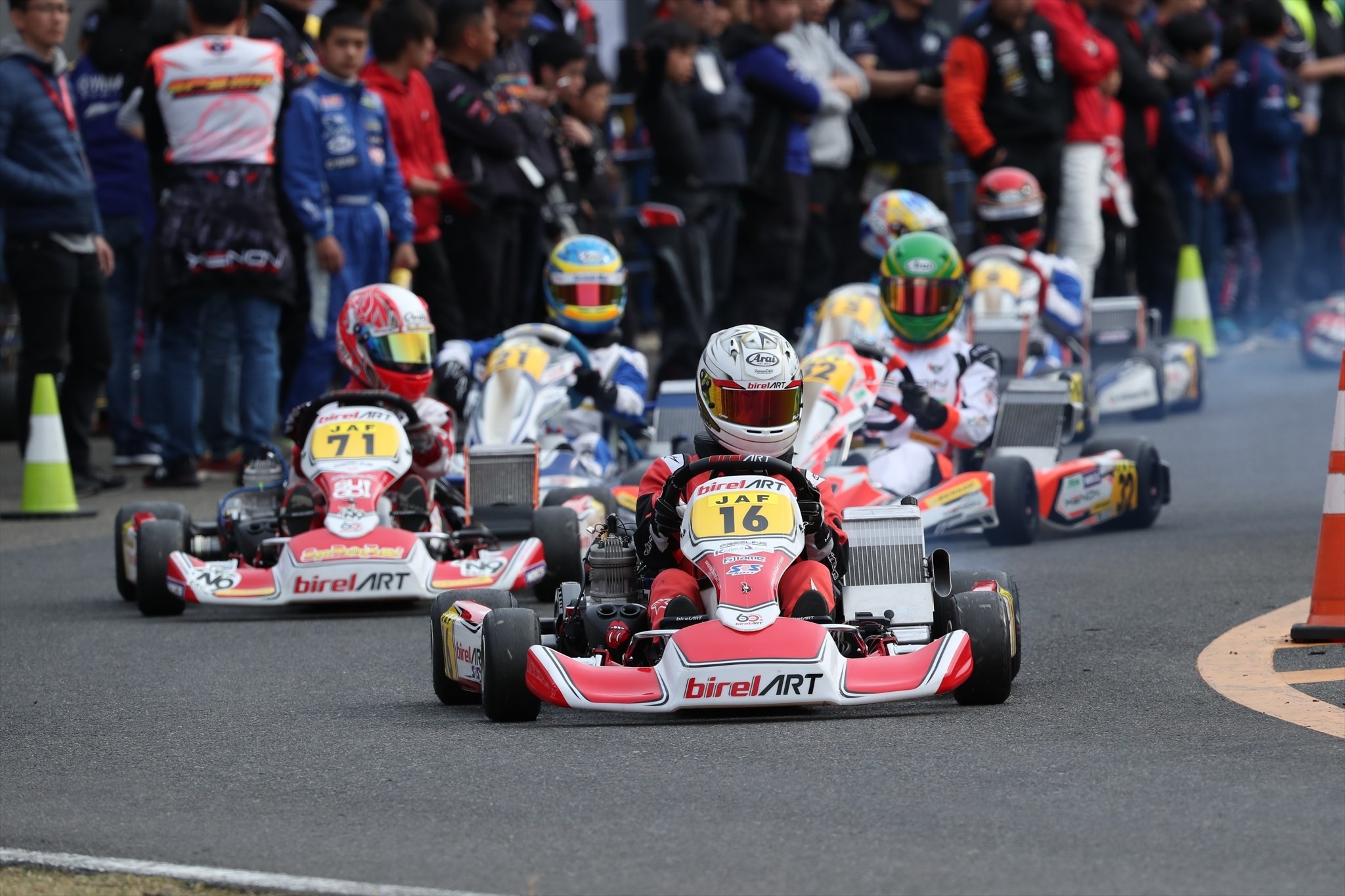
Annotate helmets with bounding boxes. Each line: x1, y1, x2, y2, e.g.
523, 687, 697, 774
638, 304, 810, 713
976, 167, 1045, 253
336, 283, 435, 401
695, 323, 803, 459
859, 189, 951, 258
878, 231, 966, 345
542, 233, 629, 334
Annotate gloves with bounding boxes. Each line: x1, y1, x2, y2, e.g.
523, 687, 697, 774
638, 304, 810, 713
798, 480, 836, 563
897, 382, 947, 429
646, 476, 685, 552
404, 421, 444, 467
284, 403, 309, 444
572, 366, 603, 403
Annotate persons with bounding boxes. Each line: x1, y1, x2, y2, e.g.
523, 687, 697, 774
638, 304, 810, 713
434, 229, 650, 500
277, 280, 458, 560
634, 322, 890, 656
962, 170, 1086, 377
0, 0, 1345, 489
797, 184, 968, 364
813, 229, 1000, 512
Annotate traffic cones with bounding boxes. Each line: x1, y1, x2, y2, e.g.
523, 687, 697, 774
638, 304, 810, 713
1168, 245, 1221, 360
1289, 348, 1345, 643
0, 373, 101, 518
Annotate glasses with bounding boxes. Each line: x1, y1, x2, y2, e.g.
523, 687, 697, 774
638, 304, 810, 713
32, 4, 72, 14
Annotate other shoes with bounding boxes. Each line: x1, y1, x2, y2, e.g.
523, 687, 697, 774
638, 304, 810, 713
113, 435, 165, 467
790, 589, 832, 624
145, 459, 203, 487
663, 595, 698, 645
73, 465, 127, 498
285, 484, 315, 535
398, 475, 429, 532
